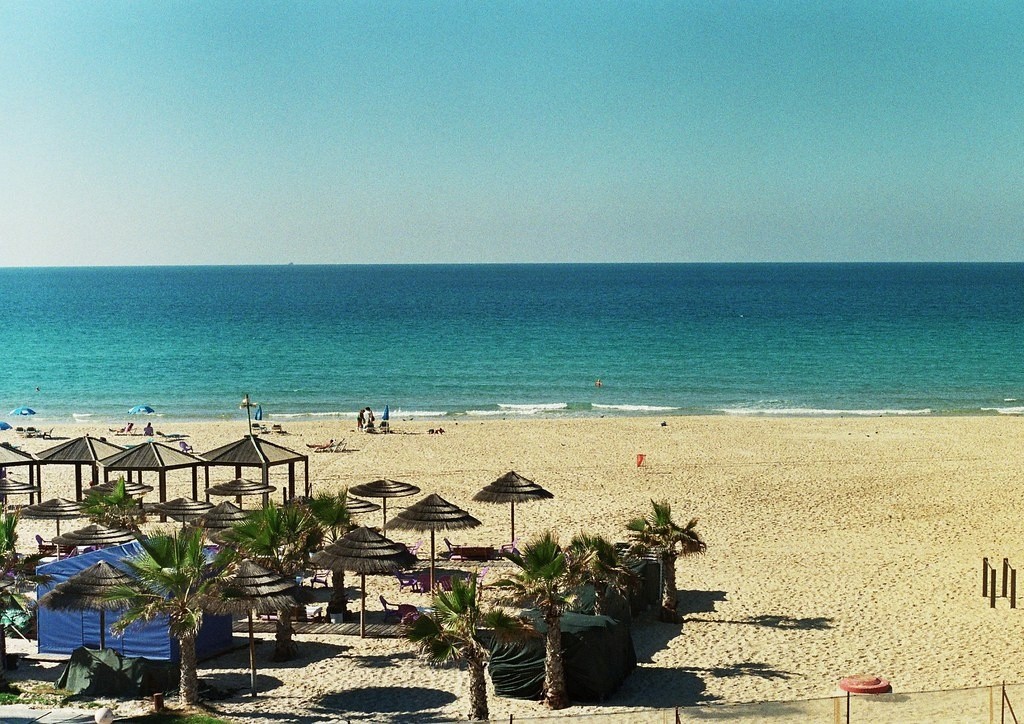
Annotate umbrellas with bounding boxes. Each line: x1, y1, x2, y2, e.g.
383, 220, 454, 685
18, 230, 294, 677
6, 407, 37, 437
38, 560, 164, 649
197, 557, 316, 698
2, 470, 554, 639
382, 404, 389, 434
0, 420, 13, 431
127, 404, 155, 436
255, 404, 262, 435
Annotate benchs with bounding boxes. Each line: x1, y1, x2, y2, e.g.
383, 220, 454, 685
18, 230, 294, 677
256, 605, 308, 620
458, 547, 496, 561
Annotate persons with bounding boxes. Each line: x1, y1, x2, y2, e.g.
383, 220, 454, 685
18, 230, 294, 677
144, 422, 153, 436
357, 407, 375, 432
109, 422, 133, 433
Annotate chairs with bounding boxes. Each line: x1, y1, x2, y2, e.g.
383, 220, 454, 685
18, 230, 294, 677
498, 535, 518, 561
379, 596, 419, 625
36, 534, 55, 553
116, 428, 137, 436
396, 568, 490, 594
315, 438, 348, 453
252, 423, 282, 434
16, 428, 54, 440
309, 571, 331, 588
179, 441, 194, 453
409, 539, 423, 556
58, 545, 103, 562
444, 539, 461, 559
363, 426, 390, 432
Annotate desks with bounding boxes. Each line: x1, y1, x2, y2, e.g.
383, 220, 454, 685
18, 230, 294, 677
306, 606, 323, 618
418, 606, 437, 614
